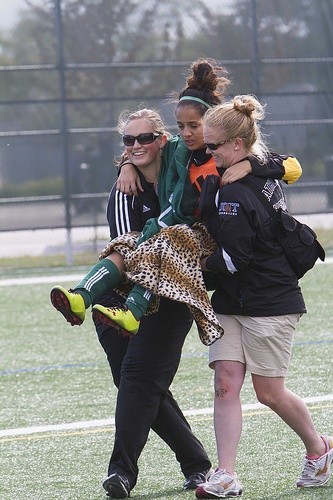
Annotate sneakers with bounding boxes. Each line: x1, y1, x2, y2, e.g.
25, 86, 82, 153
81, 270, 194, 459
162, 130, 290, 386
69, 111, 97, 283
195, 467, 244, 499
91, 303, 141, 338
296, 435, 333, 487
49, 285, 85, 326
102, 472, 130, 497
182, 466, 215, 490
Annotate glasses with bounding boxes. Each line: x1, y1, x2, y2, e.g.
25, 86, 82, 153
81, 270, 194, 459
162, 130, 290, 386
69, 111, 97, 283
203, 138, 235, 150
122, 132, 161, 146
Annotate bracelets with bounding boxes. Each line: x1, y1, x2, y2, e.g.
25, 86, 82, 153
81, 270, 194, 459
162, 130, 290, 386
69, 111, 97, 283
121, 162, 134, 167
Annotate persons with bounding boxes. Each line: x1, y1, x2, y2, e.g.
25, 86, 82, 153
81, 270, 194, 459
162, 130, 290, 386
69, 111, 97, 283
91, 109, 215, 499
195, 94, 333, 500
50, 56, 303, 341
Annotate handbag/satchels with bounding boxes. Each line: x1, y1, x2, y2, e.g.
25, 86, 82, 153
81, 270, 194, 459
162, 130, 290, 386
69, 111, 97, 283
238, 178, 326, 278
